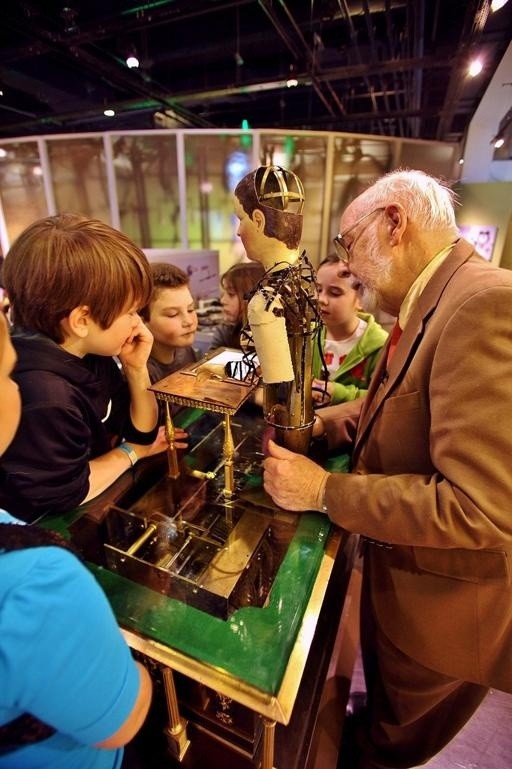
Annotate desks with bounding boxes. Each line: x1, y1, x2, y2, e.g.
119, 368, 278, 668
20, 406, 355, 767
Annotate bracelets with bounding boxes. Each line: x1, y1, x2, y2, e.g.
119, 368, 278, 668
115, 442, 139, 467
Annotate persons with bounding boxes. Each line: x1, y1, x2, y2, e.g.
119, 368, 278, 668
234, 164, 307, 386
260, 169, 512, 768
0, 211, 191, 516
0, 312, 154, 769
307, 251, 392, 407
121, 262, 202, 420
210, 260, 264, 352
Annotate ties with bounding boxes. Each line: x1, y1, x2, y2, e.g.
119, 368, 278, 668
384, 318, 403, 370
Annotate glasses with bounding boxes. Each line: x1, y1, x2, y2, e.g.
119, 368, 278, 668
332, 207, 388, 262
